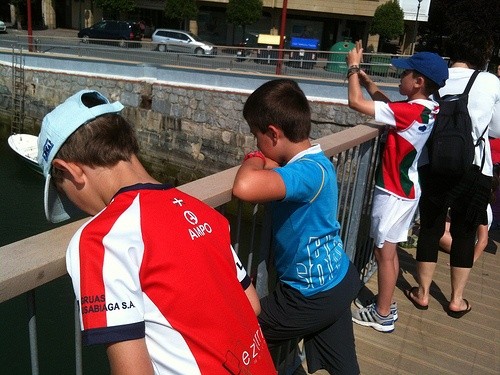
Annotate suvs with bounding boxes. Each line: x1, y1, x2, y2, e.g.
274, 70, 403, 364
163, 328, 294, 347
77, 19, 143, 48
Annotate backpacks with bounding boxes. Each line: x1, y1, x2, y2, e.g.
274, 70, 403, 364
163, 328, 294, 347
418, 71, 488, 209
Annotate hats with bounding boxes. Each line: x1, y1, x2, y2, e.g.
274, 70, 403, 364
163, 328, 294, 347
37, 90, 124, 223
391, 51, 449, 87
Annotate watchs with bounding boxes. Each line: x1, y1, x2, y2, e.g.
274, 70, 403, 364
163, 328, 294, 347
244, 151, 266, 169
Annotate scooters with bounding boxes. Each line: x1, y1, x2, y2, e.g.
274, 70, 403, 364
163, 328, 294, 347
236, 37, 250, 62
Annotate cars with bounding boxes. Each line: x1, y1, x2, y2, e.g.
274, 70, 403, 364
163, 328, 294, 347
0, 20, 7, 32
152, 28, 214, 57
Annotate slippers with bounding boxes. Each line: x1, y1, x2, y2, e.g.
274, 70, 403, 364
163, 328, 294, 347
403, 287, 428, 311
447, 299, 472, 319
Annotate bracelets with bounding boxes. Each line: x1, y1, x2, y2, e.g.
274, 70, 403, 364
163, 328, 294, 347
347, 64, 360, 78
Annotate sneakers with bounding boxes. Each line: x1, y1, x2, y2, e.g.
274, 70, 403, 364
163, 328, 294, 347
351, 304, 394, 332
355, 297, 398, 323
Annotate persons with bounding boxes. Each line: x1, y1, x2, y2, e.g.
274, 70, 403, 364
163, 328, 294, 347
405, 24, 500, 319
36, 89, 277, 375
232, 78, 360, 375
345, 48, 449, 333
